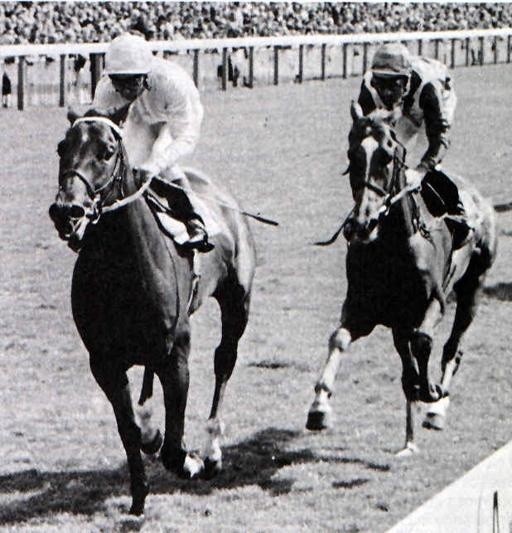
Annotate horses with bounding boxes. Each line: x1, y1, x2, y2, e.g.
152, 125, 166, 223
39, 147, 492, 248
44, 99, 260, 524
303, 98, 505, 454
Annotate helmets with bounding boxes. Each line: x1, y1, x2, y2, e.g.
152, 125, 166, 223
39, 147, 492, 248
368, 42, 414, 76
103, 35, 154, 75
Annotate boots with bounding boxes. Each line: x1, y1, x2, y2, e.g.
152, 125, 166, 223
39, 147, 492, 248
167, 175, 214, 252
444, 187, 471, 227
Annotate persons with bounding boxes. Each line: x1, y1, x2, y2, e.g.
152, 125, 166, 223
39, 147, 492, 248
89, 34, 216, 254
356, 43, 484, 248
1, 0, 512, 108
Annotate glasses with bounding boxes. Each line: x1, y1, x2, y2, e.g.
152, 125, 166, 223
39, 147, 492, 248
109, 76, 146, 91
370, 76, 403, 89
